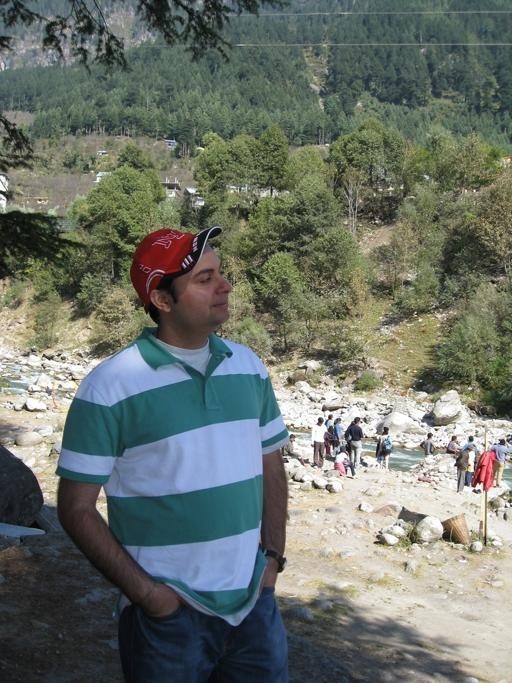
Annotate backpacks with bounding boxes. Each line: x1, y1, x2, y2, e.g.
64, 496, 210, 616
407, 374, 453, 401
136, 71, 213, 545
382, 436, 392, 453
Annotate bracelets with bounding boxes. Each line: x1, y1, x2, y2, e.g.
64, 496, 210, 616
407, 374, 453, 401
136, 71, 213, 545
262, 548, 288, 573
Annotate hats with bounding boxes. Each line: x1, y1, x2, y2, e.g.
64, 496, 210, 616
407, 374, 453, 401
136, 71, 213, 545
130, 226, 223, 308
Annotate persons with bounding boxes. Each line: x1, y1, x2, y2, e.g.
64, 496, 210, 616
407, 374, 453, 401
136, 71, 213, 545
324, 413, 512, 494
281, 432, 310, 467
310, 416, 328, 468
57, 226, 295, 683
